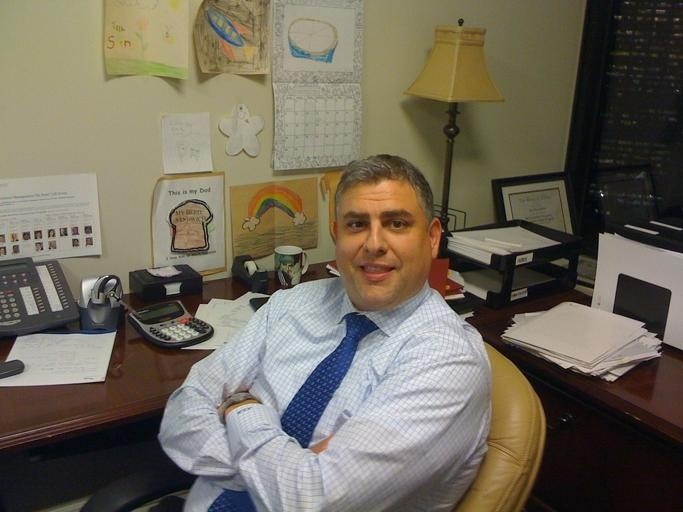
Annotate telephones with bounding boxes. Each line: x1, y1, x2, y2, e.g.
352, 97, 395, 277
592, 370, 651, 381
0, 258, 81, 335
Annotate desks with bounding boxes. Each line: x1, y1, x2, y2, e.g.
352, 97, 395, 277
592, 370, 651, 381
443, 289, 683, 510
0, 258, 338, 456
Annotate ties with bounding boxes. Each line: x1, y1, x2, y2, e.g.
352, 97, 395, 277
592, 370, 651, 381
207, 307, 380, 509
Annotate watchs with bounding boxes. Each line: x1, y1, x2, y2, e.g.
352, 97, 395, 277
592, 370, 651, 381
215, 390, 263, 425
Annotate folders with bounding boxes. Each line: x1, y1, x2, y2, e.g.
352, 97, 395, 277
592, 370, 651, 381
590, 218, 682, 351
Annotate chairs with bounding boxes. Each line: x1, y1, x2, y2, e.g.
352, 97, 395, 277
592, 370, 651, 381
80, 339, 549, 512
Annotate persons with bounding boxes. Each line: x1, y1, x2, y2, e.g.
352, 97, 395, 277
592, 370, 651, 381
153, 152, 493, 512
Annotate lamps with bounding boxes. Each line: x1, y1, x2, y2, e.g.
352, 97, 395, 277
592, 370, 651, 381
401, 15, 506, 259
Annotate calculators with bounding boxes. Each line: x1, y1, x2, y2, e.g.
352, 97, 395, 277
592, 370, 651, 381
127, 299, 213, 350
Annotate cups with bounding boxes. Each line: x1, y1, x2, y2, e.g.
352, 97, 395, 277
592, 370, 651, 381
274, 246, 308, 290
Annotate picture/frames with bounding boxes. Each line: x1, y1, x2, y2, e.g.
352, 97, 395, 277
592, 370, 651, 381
491, 172, 582, 236
583, 164, 659, 233
